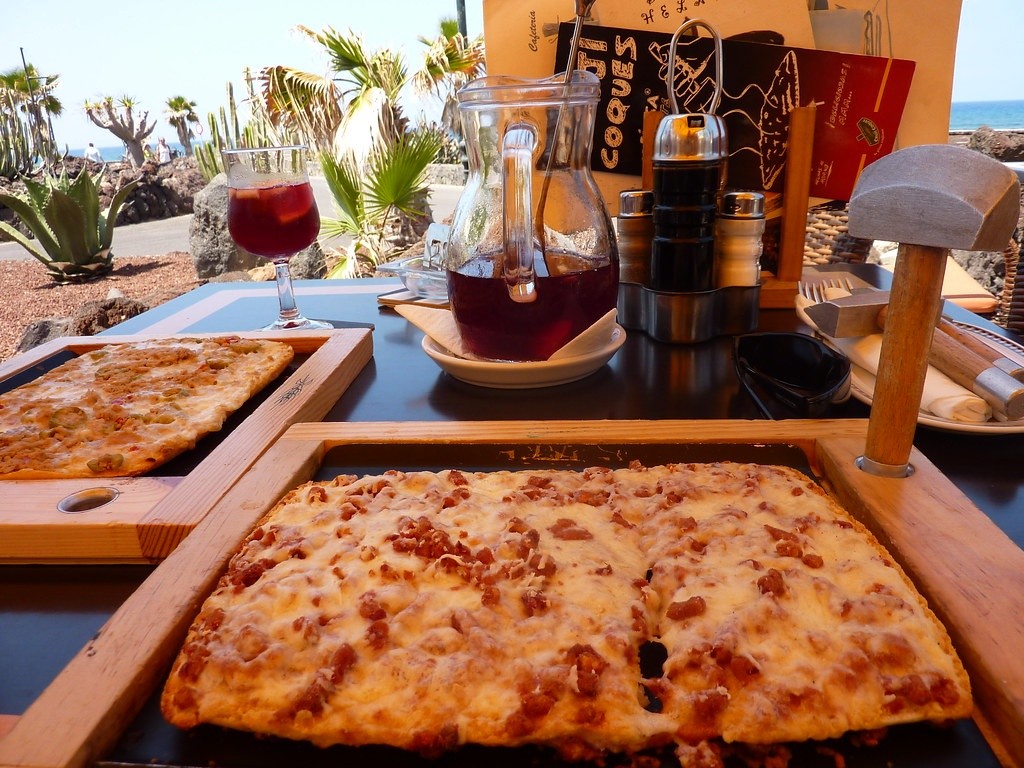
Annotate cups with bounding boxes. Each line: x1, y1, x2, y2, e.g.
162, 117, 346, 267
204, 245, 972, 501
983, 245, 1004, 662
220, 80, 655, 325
221, 146, 333, 329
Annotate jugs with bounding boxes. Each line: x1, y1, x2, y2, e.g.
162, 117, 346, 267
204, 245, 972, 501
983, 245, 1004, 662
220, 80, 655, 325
446, 71, 620, 363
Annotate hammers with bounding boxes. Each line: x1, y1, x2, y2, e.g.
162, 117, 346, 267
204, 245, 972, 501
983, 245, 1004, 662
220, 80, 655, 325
803, 286, 1024, 424
849, 144, 1021, 480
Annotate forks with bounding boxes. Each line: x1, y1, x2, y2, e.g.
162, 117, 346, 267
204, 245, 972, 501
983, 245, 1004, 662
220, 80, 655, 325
798, 278, 854, 304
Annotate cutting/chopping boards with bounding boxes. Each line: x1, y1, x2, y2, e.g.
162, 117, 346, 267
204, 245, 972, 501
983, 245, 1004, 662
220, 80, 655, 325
0, 419, 1022, 768
0, 328, 377, 559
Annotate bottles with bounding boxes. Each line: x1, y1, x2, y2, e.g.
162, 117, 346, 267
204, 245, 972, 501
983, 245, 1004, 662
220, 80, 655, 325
618, 189, 653, 281
716, 192, 766, 286
652, 114, 728, 291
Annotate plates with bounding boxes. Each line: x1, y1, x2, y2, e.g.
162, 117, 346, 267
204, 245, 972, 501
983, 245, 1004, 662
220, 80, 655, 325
421, 324, 628, 390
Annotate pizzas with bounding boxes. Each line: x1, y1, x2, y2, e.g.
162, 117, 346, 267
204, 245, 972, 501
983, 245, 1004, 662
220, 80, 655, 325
0, 335, 294, 481
160, 460, 973, 761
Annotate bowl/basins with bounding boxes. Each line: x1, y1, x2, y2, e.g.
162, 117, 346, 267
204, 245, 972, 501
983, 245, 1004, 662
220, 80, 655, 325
375, 254, 450, 300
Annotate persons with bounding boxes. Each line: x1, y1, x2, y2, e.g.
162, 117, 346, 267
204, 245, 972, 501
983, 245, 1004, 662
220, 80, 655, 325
143, 144, 156, 161
240, 133, 245, 149
84, 143, 105, 163
156, 136, 173, 165
